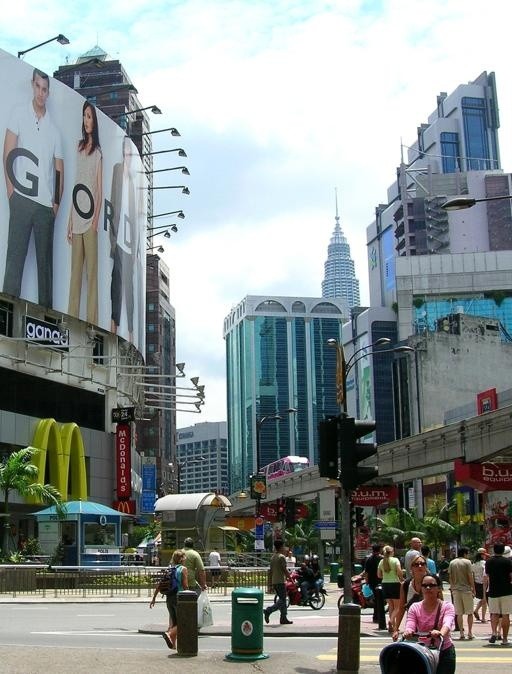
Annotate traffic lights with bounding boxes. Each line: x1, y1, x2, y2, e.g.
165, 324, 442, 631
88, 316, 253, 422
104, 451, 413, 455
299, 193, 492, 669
360, 507, 365, 525
277, 497, 285, 521
294, 502, 301, 522
287, 497, 294, 526
358, 418, 382, 484
351, 504, 356, 526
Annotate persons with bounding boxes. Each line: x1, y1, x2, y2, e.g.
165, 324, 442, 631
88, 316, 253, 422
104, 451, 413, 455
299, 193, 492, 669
208, 548, 222, 588
263, 537, 512, 674
150, 551, 188, 649
67, 101, 102, 326
2, 69, 64, 309
110, 135, 136, 344
171, 537, 208, 597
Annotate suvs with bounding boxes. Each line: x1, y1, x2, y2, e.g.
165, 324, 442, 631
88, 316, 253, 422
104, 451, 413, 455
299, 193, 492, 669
121, 553, 144, 567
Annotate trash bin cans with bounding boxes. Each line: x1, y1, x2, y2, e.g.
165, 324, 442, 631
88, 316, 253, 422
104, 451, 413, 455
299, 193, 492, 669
225, 588, 269, 660
329, 563, 339, 583
354, 564, 363, 575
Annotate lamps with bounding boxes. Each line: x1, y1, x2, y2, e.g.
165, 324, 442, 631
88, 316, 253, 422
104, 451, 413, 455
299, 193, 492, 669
14, 33, 191, 255
1, 312, 205, 415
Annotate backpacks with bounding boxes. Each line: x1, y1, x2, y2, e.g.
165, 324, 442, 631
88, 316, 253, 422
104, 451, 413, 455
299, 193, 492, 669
160, 564, 182, 595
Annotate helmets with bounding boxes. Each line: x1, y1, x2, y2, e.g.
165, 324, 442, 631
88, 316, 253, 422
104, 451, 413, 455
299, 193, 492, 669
311, 554, 320, 564
303, 555, 310, 564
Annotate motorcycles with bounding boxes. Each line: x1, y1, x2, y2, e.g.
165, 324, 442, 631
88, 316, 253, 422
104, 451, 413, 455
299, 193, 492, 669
275, 568, 328, 610
339, 572, 390, 617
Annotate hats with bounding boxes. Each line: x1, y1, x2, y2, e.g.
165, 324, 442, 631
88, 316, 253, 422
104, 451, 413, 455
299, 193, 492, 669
502, 546, 512, 558
477, 548, 490, 556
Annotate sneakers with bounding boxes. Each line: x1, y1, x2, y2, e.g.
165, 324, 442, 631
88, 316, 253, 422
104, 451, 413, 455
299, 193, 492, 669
501, 643, 512, 648
163, 632, 174, 649
489, 636, 496, 643
264, 610, 269, 623
280, 618, 293, 624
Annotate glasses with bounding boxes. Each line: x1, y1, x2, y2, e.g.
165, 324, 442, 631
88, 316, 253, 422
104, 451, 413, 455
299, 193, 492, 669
411, 561, 426, 568
421, 583, 438, 588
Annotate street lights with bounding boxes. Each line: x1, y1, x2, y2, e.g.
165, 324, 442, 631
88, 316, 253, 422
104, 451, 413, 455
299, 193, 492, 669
241, 406, 300, 561
166, 454, 205, 494
326, 335, 417, 412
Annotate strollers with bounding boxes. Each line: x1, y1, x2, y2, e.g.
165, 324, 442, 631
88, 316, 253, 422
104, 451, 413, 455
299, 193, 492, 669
380, 632, 444, 673
